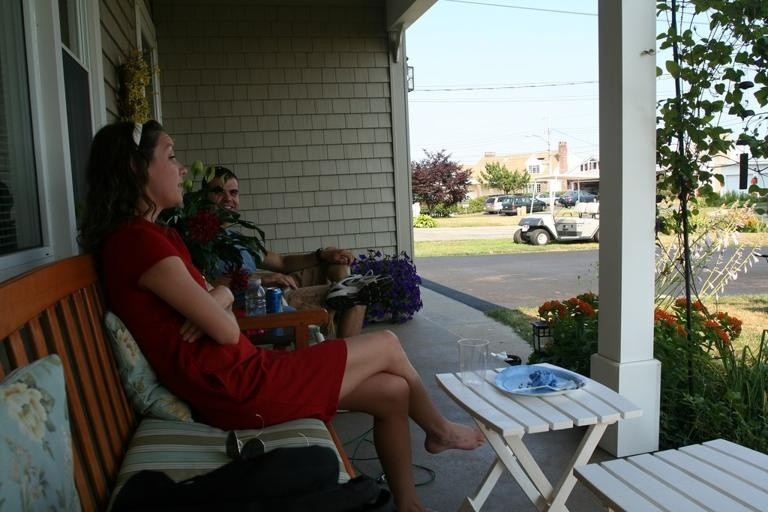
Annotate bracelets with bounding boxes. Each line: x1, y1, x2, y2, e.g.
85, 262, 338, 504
315, 246, 323, 265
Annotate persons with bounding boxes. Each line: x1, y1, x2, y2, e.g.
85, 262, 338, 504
73, 116, 486, 512
197, 163, 395, 340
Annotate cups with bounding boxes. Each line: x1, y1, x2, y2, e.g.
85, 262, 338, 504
457, 338, 491, 386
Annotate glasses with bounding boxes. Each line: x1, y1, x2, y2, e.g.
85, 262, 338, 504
225, 413, 310, 461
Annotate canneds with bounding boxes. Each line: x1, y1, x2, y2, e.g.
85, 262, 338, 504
266, 287, 283, 315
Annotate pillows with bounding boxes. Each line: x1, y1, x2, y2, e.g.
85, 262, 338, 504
0, 353, 82, 512
105, 312, 192, 423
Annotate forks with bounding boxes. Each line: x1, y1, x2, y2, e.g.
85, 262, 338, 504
508, 383, 567, 391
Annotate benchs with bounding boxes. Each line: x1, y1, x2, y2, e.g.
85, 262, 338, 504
0, 253, 356, 512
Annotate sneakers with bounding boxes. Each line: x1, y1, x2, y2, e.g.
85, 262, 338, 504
326, 272, 394, 311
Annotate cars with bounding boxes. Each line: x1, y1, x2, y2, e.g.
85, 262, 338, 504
483, 188, 598, 215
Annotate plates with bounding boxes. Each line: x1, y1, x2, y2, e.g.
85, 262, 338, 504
495, 365, 586, 397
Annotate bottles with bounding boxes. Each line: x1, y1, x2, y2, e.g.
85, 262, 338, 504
243, 278, 266, 335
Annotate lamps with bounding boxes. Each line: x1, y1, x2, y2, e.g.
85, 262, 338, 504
530, 321, 553, 354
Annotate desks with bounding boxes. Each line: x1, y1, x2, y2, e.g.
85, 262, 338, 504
435, 361, 643, 512
573, 437, 768, 512
